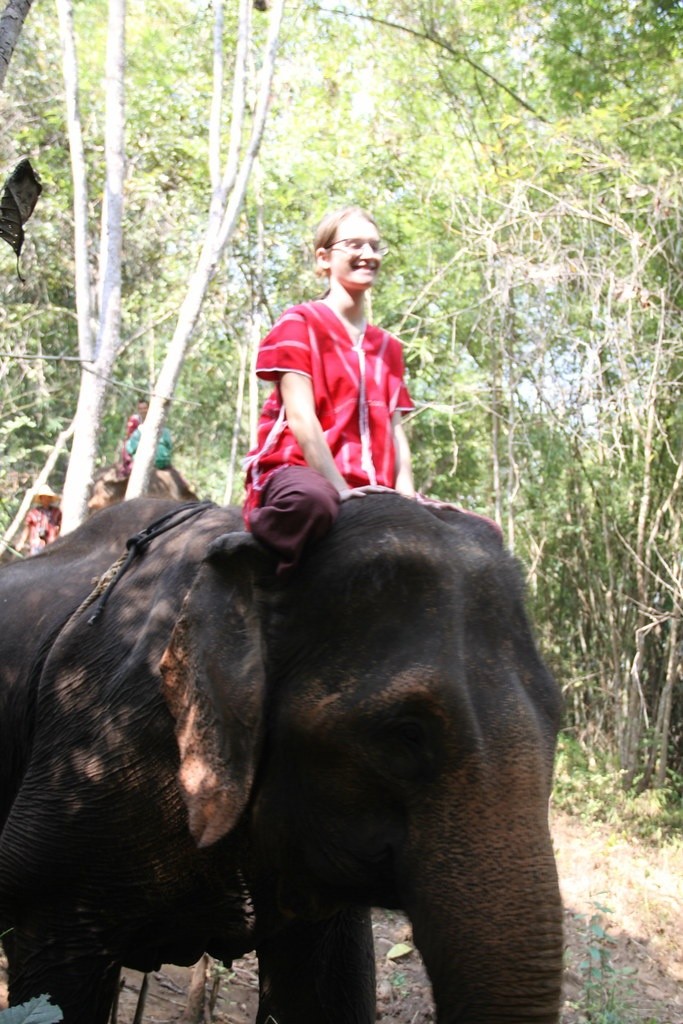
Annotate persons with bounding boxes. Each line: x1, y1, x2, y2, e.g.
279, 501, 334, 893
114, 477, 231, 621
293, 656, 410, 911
14, 482, 62, 558
115, 399, 149, 477
240, 204, 418, 573
126, 421, 174, 469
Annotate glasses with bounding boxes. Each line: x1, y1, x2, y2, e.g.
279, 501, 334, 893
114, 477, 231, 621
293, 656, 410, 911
325, 238, 389, 256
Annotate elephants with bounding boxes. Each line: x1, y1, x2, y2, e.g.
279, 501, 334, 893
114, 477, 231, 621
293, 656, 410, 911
0, 490, 564, 1024
85, 466, 201, 516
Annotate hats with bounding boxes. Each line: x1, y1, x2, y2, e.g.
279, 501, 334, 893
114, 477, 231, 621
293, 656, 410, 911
34, 485, 59, 504
